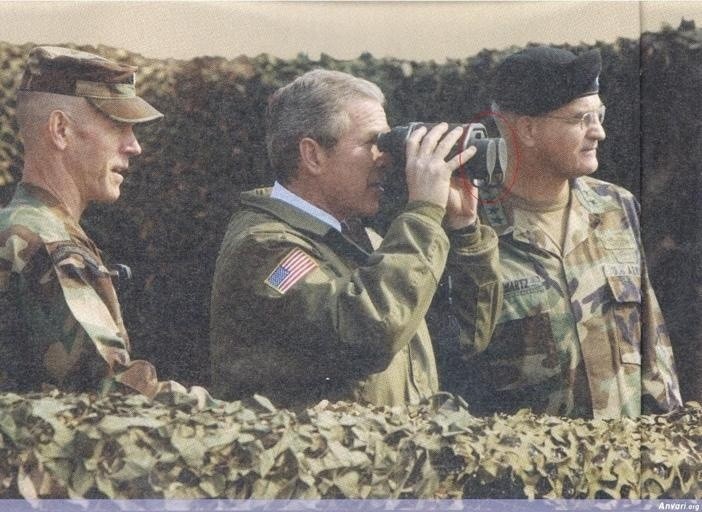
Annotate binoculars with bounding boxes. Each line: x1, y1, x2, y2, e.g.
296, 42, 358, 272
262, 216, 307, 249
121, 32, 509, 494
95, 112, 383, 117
376, 123, 511, 187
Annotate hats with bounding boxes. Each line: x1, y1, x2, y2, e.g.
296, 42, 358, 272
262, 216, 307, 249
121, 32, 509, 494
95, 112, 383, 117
489, 47, 602, 115
19, 46, 164, 127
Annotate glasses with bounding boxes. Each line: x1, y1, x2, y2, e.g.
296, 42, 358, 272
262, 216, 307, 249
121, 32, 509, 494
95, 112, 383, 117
547, 104, 606, 130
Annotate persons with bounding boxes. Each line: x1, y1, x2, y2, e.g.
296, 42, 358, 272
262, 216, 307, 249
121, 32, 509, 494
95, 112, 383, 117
210, 68, 502, 413
0, 46, 232, 412
438, 48, 685, 422
